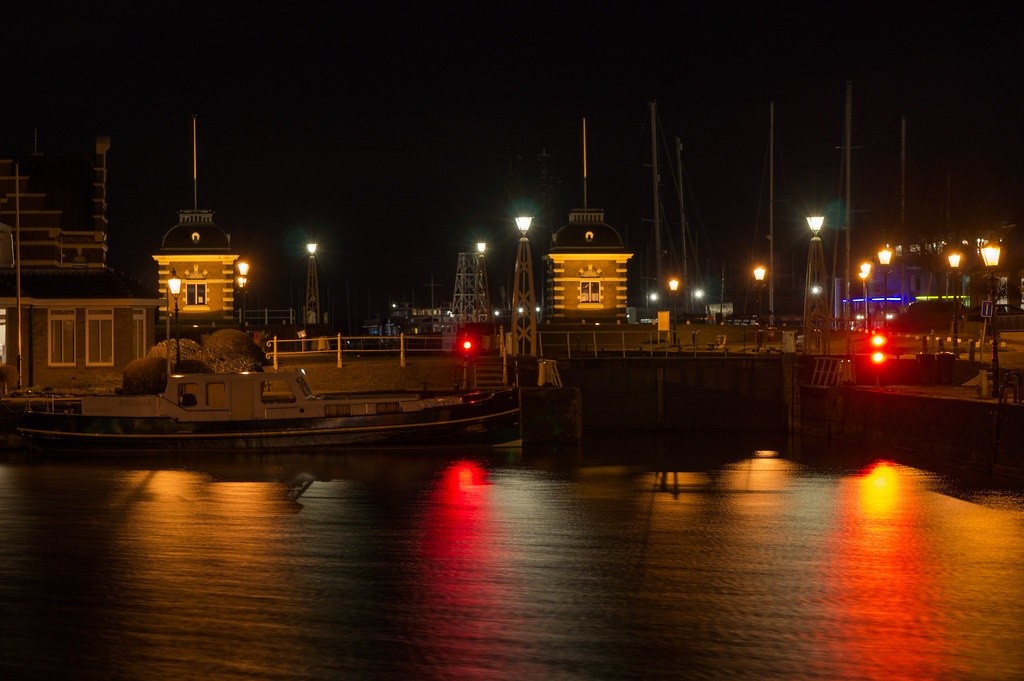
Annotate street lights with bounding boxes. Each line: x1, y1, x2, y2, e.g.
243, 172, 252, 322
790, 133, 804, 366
239, 261, 249, 321
668, 276, 681, 348
304, 241, 319, 325
166, 267, 182, 375
508, 215, 537, 358
474, 241, 492, 323
861, 263, 871, 332
946, 251, 963, 355
981, 242, 1001, 400
801, 214, 831, 354
878, 250, 892, 332
753, 266, 768, 325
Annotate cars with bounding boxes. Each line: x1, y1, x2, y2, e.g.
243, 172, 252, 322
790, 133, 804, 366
964, 303, 1024, 333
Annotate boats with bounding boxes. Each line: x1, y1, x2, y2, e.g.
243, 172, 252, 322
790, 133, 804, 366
13, 368, 523, 456
